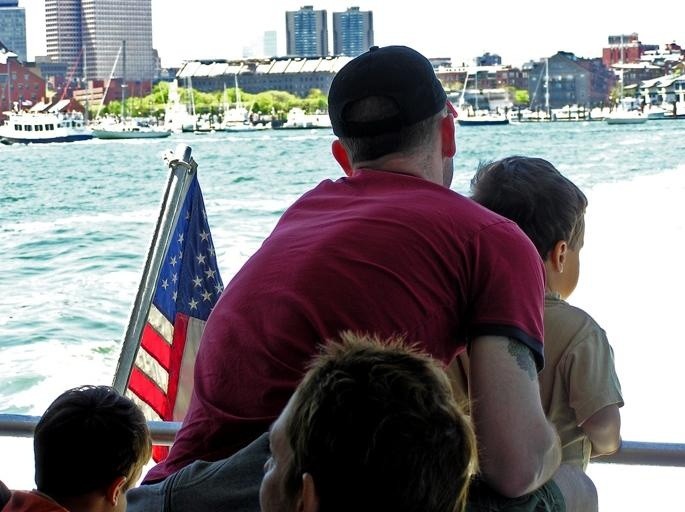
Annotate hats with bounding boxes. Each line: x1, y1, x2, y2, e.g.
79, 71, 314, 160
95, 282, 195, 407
328, 44, 458, 139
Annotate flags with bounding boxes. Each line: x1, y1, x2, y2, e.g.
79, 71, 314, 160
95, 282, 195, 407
124, 172, 224, 464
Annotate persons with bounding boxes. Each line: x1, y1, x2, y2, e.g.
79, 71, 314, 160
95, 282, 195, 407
1, 384, 155, 512
467, 155, 626, 471
137, 43, 599, 511
257, 328, 481, 512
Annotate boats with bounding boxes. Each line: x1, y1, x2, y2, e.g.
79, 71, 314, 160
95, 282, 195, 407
457, 116, 509, 125
605, 111, 647, 123
0, 109, 171, 144
